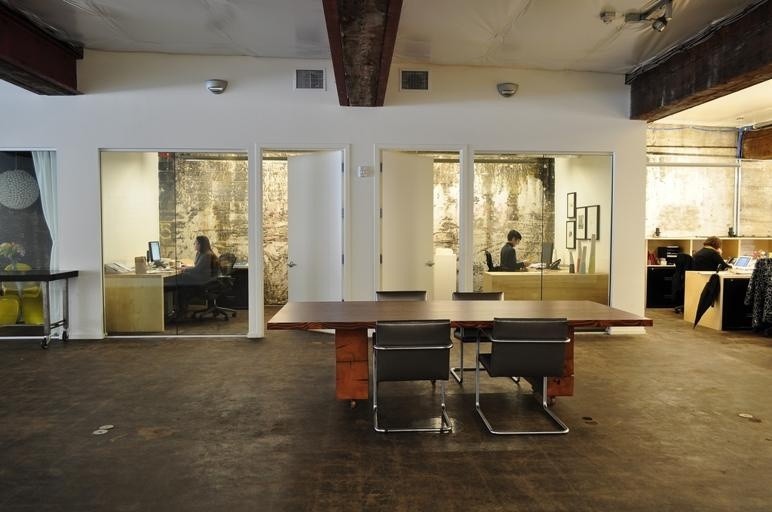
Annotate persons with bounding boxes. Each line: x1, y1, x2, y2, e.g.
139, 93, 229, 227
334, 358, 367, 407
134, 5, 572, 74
692, 236, 732, 271
172, 236, 221, 321
500, 230, 531, 272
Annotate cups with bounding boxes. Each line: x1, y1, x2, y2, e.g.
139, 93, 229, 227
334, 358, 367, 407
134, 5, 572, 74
660, 260, 667, 265
176, 260, 184, 269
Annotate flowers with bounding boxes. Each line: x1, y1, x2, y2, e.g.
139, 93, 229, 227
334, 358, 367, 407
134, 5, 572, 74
0, 242, 25, 258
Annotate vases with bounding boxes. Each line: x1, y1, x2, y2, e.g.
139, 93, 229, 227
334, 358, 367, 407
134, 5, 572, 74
11, 260, 17, 270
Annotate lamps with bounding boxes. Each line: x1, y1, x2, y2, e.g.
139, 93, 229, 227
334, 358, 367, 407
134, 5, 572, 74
204, 79, 229, 93
497, 83, 518, 98
653, 0, 673, 31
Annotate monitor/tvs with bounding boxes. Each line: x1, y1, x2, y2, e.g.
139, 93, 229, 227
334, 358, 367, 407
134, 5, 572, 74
541, 242, 554, 268
731, 255, 753, 268
148, 241, 168, 268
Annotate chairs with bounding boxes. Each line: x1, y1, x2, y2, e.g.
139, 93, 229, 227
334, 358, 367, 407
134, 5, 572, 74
373, 290, 571, 435
189, 253, 237, 321
485, 250, 494, 272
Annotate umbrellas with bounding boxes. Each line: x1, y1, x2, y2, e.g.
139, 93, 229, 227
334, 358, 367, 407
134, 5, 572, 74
693, 264, 722, 329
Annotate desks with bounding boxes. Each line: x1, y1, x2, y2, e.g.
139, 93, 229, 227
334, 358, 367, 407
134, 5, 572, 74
266, 299, 653, 407
484, 267, 608, 304
647, 238, 772, 330
104, 267, 184, 333
0, 269, 78, 347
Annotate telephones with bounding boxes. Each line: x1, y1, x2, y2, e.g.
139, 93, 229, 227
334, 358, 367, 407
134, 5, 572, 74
551, 259, 561, 269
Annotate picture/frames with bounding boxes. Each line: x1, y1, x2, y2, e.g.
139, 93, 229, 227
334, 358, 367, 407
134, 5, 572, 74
565, 192, 600, 249
